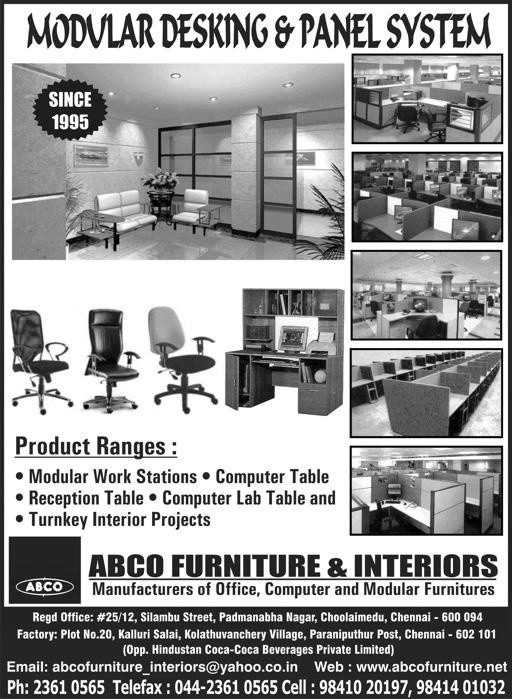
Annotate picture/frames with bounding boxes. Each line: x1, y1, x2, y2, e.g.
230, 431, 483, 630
72, 142, 111, 170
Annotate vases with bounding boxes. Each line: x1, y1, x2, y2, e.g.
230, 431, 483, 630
154, 186, 169, 193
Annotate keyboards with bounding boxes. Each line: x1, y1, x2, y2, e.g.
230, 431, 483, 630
386, 501, 400, 504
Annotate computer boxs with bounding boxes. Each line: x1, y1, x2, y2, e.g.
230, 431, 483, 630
239, 363, 249, 394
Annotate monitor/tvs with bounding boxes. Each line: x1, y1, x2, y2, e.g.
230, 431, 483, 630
387, 483, 401, 500
277, 326, 309, 355
383, 294, 391, 301
371, 292, 376, 296
452, 219, 480, 241
463, 294, 471, 301
413, 298, 427, 311
394, 205, 413, 224
388, 173, 501, 202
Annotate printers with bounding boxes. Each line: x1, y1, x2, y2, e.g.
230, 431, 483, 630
306, 332, 337, 355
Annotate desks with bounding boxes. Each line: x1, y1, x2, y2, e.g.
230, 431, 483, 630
352, 290, 499, 339
352, 169, 503, 241
350, 468, 502, 535
353, 72, 500, 143
350, 348, 503, 438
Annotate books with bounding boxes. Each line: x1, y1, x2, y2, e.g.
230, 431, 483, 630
301, 361, 307, 383
305, 366, 314, 383
270, 290, 287, 315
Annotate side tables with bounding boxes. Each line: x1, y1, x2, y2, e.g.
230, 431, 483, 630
80, 210, 123, 251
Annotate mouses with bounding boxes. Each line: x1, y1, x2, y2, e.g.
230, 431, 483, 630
404, 502, 407, 505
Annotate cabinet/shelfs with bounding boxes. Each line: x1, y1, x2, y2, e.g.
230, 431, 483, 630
223, 289, 343, 417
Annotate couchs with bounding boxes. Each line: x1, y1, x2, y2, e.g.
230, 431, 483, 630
96, 189, 158, 245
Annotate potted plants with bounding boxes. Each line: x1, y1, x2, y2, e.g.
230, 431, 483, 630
66, 174, 85, 258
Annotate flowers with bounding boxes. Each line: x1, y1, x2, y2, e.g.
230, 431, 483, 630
143, 168, 176, 187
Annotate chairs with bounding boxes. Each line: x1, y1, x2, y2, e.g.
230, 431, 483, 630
10, 303, 219, 414
172, 187, 210, 236
